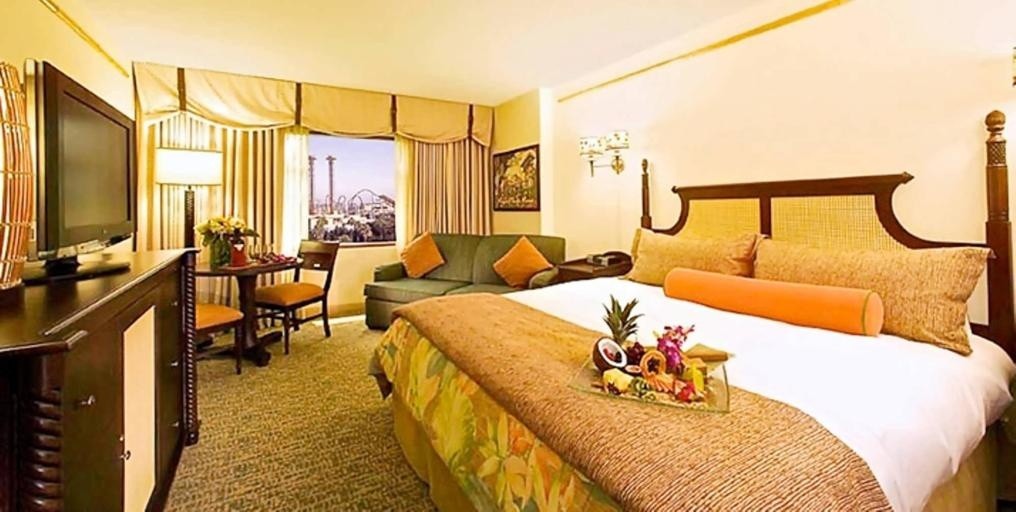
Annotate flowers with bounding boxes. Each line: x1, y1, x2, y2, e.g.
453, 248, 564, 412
191, 213, 263, 247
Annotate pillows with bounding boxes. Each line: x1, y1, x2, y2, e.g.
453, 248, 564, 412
660, 263, 886, 337
617, 225, 770, 291
751, 232, 998, 358
492, 234, 556, 290
397, 231, 446, 280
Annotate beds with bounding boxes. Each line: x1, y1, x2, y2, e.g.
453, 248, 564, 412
362, 104, 1016, 512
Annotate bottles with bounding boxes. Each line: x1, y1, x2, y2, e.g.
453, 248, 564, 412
230, 228, 245, 267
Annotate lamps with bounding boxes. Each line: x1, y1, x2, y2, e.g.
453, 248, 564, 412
152, 146, 223, 351
1012, 44, 1016, 93
577, 124, 632, 179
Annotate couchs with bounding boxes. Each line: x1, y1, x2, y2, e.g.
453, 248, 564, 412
360, 230, 567, 331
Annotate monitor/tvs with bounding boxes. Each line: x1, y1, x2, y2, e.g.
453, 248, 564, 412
21, 58, 137, 286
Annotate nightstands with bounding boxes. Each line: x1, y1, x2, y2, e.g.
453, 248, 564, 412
555, 251, 632, 288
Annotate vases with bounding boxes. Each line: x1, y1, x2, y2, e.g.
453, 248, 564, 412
205, 238, 231, 271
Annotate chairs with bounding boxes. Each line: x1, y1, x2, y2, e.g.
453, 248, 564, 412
177, 261, 245, 389
237, 237, 340, 355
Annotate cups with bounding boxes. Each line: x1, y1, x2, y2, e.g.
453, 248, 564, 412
249, 244, 273, 260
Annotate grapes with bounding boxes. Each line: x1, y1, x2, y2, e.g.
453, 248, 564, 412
626, 342, 644, 364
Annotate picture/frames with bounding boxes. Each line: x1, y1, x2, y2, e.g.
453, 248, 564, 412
491, 141, 541, 213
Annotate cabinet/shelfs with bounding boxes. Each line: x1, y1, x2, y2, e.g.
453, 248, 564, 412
1, 237, 204, 512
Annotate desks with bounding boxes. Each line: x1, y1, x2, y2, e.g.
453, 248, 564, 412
189, 252, 306, 375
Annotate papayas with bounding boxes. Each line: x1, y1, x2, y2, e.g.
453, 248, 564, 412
640, 349, 667, 377
